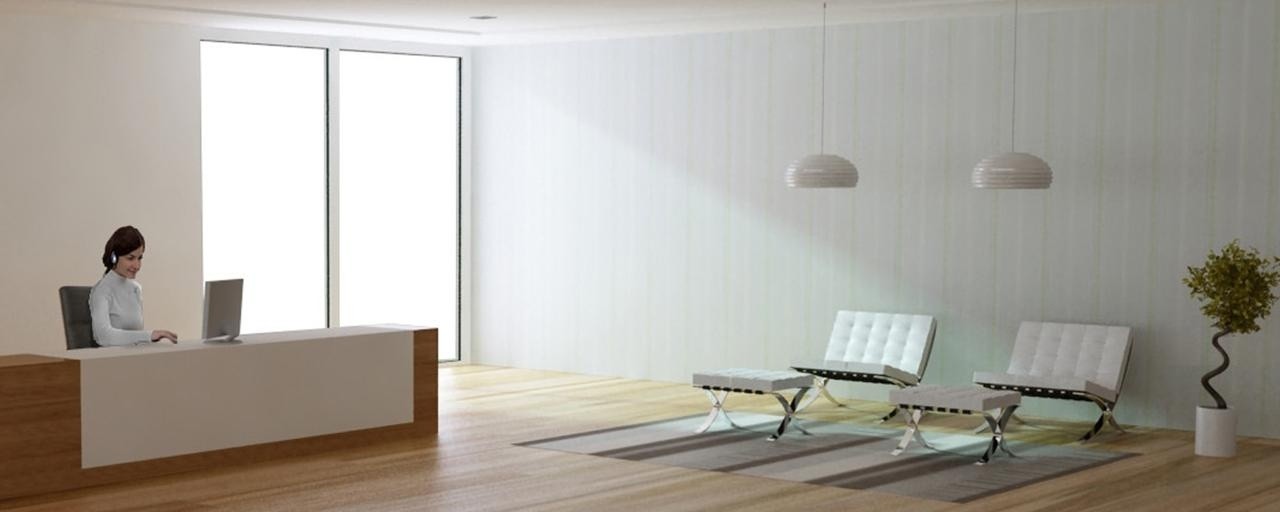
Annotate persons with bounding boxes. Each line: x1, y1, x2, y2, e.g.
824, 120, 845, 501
89, 225, 178, 345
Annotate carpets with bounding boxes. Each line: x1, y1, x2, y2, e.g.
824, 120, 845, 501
511, 409, 1141, 503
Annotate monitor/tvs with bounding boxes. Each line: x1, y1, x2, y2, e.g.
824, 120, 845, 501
200, 278, 245, 345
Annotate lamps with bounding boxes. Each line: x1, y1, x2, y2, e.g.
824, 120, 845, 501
971, 0, 1054, 190
787, 1, 858, 189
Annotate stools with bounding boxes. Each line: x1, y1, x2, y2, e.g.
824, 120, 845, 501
692, 367, 814, 442
889, 384, 1023, 466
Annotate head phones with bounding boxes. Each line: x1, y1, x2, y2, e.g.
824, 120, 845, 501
109, 252, 118, 269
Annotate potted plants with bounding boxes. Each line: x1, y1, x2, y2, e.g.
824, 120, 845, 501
1183, 238, 1279, 460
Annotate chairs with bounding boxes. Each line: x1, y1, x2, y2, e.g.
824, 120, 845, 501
972, 321, 1134, 445
788, 311, 937, 425
59, 286, 101, 349
